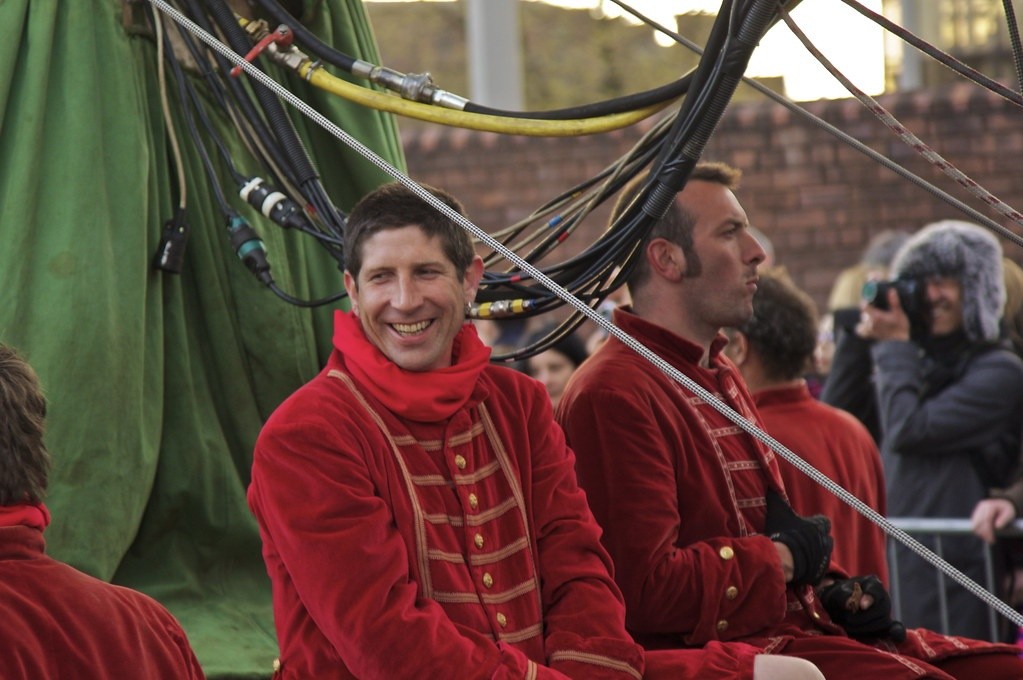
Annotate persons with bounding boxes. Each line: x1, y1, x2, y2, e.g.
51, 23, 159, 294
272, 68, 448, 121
553, 164, 1023, 680
245, 181, 825, 680
812, 218, 1023, 642
719, 273, 890, 592
971, 480, 1023, 542
0, 342, 206, 680
522, 320, 589, 406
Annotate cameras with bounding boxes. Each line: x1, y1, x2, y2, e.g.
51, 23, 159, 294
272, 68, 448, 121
862, 272, 930, 340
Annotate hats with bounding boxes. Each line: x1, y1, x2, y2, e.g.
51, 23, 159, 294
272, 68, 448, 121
867, 227, 910, 262
890, 221, 1007, 344
830, 264, 862, 309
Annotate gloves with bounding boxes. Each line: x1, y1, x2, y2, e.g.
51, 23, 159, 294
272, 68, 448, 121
769, 512, 833, 590
817, 572, 893, 646
